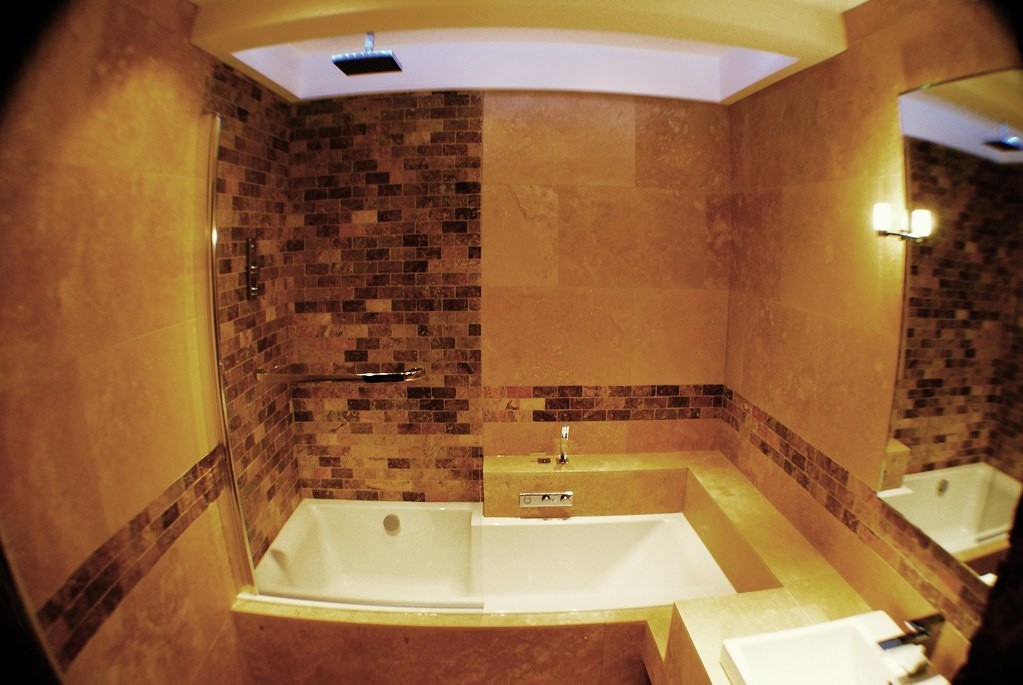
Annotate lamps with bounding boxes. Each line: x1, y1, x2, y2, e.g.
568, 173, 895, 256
909, 207, 932, 241
871, 200, 905, 238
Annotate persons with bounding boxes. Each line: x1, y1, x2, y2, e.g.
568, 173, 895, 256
950, 490, 1023, 685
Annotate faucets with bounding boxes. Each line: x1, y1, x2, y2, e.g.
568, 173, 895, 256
876, 613, 945, 684
555, 425, 570, 465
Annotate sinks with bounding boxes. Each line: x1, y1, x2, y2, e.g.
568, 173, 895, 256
724, 607, 956, 685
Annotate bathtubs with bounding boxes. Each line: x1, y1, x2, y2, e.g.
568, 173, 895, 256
875, 456, 1023, 558
254, 499, 738, 617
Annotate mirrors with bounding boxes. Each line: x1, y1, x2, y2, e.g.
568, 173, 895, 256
876, 67, 1023, 585
